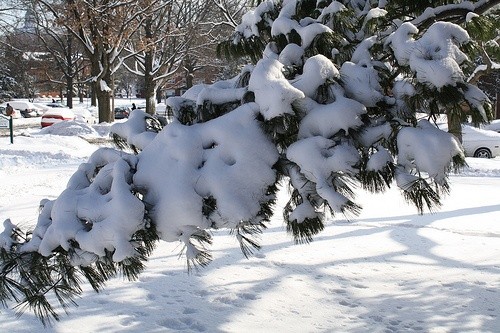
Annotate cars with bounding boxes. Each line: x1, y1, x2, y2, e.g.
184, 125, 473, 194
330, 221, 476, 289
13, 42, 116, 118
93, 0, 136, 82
40, 108, 78, 127
0, 100, 70, 117
437, 123, 500, 158
112, 106, 131, 119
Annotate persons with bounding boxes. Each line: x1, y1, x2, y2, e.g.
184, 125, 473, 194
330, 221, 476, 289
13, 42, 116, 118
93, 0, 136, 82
6, 103, 14, 116
131, 103, 136, 111
52, 99, 55, 103
